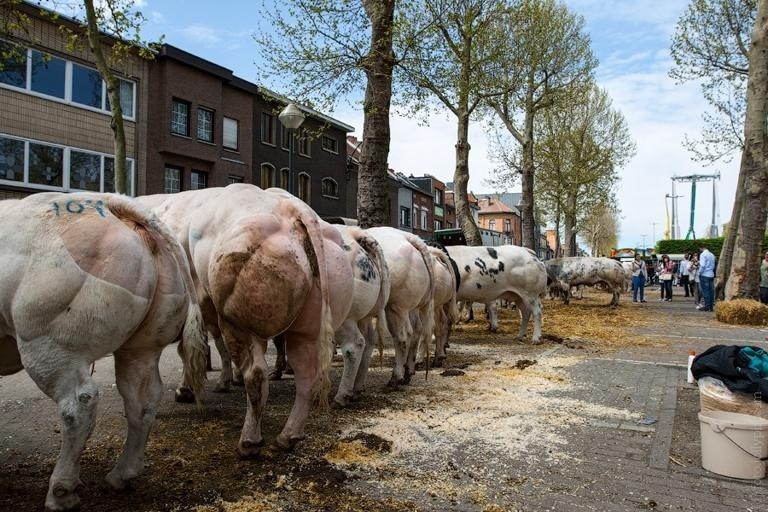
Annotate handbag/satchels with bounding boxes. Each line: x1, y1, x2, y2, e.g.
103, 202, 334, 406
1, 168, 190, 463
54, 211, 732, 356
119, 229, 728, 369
658, 273, 672, 283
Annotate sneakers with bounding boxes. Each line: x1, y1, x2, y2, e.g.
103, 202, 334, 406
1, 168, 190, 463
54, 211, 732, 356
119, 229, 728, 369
695, 303, 713, 312
632, 300, 646, 302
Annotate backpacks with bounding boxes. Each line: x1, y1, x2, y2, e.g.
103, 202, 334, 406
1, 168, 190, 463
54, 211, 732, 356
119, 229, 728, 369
690, 345, 768, 402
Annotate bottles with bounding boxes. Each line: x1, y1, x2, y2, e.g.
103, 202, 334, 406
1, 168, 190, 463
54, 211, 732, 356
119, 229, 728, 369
687, 350, 696, 384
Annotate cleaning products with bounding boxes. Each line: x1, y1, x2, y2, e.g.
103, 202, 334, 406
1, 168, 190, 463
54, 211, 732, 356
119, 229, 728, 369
688, 351, 695, 383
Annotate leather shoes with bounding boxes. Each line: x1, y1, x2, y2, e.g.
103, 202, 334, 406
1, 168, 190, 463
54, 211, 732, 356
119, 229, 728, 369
658, 298, 672, 302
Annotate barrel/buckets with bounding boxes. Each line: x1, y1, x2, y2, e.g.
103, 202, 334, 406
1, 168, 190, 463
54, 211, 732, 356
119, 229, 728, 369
698, 411, 768, 480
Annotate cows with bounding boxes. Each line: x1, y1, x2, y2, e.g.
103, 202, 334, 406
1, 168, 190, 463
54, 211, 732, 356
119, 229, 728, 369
0, 187, 193, 509
130, 183, 546, 469
540, 257, 632, 309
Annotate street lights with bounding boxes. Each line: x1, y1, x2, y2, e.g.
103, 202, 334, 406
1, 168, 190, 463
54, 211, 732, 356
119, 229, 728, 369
278, 98, 307, 202
571, 223, 579, 259
514, 197, 529, 252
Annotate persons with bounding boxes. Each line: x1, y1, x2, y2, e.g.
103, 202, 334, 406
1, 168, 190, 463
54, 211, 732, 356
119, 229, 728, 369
759, 250, 767, 303
631, 253, 648, 303
657, 243, 716, 312
